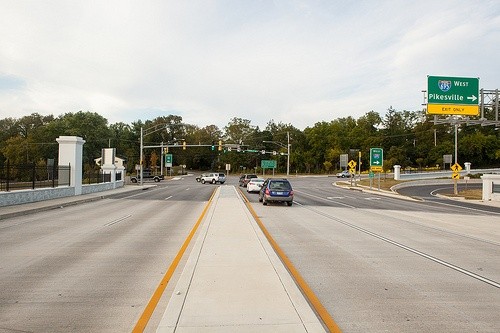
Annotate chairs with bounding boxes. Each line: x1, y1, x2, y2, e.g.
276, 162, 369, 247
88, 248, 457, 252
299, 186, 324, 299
279, 184, 284, 187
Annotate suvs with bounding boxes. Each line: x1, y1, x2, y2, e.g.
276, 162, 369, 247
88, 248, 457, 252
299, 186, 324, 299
258, 177, 294, 206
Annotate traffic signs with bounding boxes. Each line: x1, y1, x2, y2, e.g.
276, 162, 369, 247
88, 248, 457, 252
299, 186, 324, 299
369, 147, 384, 172
426, 74, 480, 117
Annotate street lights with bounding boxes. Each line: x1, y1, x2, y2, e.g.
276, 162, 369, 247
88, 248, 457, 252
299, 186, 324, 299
160, 138, 186, 176
262, 132, 290, 176
140, 125, 170, 186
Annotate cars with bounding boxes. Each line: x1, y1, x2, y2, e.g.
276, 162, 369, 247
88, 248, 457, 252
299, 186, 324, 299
245, 178, 265, 193
336, 170, 351, 178
195, 172, 226, 184
238, 173, 257, 187
131, 172, 164, 182
